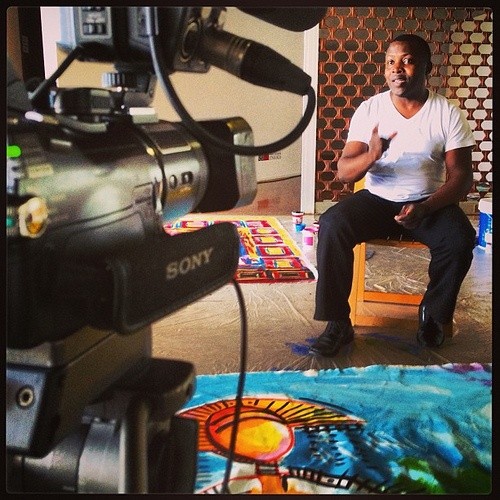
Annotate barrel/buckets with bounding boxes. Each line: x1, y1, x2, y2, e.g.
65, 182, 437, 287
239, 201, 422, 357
292, 211, 303, 225
477, 198, 493, 250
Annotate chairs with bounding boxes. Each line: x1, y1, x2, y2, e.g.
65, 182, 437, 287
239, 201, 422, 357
348, 178, 427, 332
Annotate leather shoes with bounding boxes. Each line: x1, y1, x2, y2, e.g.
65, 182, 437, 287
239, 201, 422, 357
309, 318, 358, 356
416, 326, 445, 349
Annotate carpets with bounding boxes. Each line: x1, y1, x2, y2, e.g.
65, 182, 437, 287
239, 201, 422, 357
176, 363, 492, 498
163, 214, 318, 284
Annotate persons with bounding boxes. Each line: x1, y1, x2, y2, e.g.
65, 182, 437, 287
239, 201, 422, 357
308, 34, 476, 358
